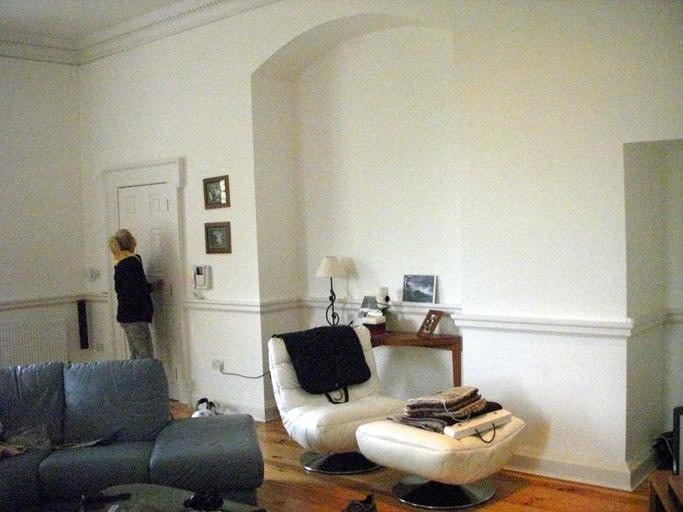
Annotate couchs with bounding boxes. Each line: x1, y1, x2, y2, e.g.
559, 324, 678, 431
353, 416, 524, 508
0, 356, 262, 512
261, 325, 403, 476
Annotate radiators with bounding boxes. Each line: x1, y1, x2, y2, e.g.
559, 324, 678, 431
0, 321, 68, 366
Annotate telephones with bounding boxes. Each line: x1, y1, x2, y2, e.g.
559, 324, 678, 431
191, 265, 211, 290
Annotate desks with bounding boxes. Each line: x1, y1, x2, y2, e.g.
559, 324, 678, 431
371, 330, 461, 388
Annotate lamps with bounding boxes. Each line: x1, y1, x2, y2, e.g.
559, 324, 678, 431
316, 254, 345, 327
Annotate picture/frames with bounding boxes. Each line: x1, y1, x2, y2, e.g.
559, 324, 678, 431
403, 273, 436, 304
201, 174, 230, 210
418, 309, 442, 338
202, 221, 231, 254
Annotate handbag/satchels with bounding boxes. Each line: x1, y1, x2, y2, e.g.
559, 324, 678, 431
268, 323, 370, 404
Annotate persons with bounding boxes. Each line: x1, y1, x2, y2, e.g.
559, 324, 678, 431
109, 228, 165, 359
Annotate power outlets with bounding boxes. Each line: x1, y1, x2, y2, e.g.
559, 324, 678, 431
210, 358, 224, 368
